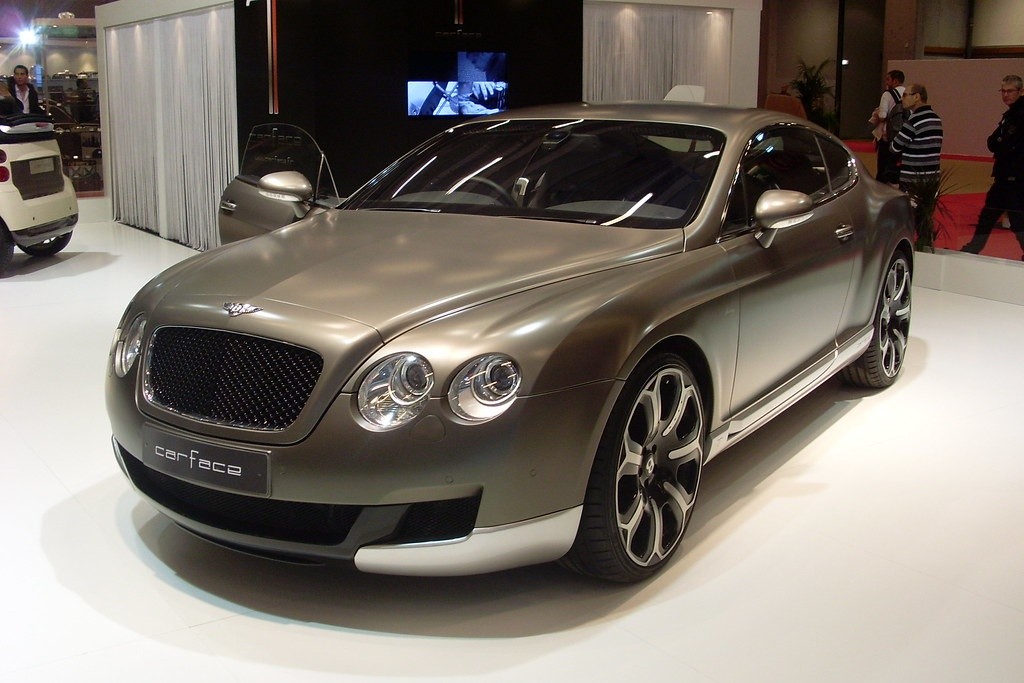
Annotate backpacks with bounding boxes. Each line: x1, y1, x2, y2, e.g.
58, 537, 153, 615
883, 89, 911, 142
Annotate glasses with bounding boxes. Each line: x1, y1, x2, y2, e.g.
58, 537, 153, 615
902, 93, 915, 98
998, 89, 1019, 94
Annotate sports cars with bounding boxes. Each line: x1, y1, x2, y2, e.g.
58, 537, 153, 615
106, 100, 917, 588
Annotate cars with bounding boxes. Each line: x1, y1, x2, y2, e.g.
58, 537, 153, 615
0, 95, 80, 271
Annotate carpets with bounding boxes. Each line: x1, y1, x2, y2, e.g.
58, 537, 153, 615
912, 191, 1024, 262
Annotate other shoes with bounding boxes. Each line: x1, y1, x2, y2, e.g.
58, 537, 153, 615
959, 244, 979, 255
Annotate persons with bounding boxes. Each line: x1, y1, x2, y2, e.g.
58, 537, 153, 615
457, 51, 508, 116
891, 83, 944, 254
70, 79, 88, 117
7, 65, 40, 116
876, 69, 913, 184
958, 75, 1024, 262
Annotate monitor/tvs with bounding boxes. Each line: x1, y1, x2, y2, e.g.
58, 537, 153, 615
404, 48, 511, 120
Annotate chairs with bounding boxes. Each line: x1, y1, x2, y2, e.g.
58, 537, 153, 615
647, 150, 748, 227
